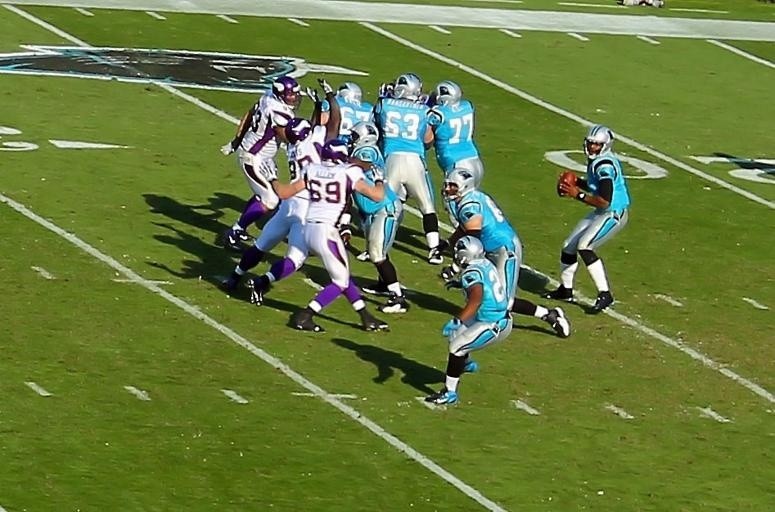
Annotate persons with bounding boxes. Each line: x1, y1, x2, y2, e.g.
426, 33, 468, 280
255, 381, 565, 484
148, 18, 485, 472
255, 139, 394, 332
421, 234, 513, 409
430, 165, 574, 341
541, 123, 633, 317
221, 61, 488, 318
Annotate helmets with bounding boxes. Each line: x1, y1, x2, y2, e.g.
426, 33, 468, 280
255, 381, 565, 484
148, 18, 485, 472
284, 117, 313, 146
272, 77, 301, 111
393, 72, 423, 102
442, 168, 475, 202
583, 126, 614, 160
454, 236, 484, 268
348, 121, 379, 148
436, 80, 462, 105
334, 82, 362, 105
320, 139, 350, 162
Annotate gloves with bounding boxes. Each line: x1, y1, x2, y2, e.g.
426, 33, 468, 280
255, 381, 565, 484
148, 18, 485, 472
306, 86, 319, 103
441, 316, 463, 338
219, 140, 236, 157
258, 162, 278, 183
317, 78, 335, 97
370, 163, 386, 184
378, 83, 388, 97
428, 239, 451, 261
441, 263, 460, 280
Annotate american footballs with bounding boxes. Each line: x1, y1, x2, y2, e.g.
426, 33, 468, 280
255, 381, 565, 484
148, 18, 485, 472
558, 171, 577, 196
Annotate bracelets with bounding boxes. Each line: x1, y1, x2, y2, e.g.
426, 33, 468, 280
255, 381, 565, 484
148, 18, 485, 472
577, 191, 586, 200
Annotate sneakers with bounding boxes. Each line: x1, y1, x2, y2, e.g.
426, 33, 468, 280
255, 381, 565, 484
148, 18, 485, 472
425, 385, 458, 406
541, 284, 573, 302
288, 309, 326, 333
224, 235, 247, 252
222, 279, 238, 292
361, 319, 388, 333
586, 291, 613, 314
247, 275, 265, 307
541, 306, 570, 337
427, 255, 445, 264
376, 291, 410, 313
357, 249, 372, 262
338, 222, 352, 250
463, 360, 477, 373
230, 222, 257, 241
361, 283, 390, 296
